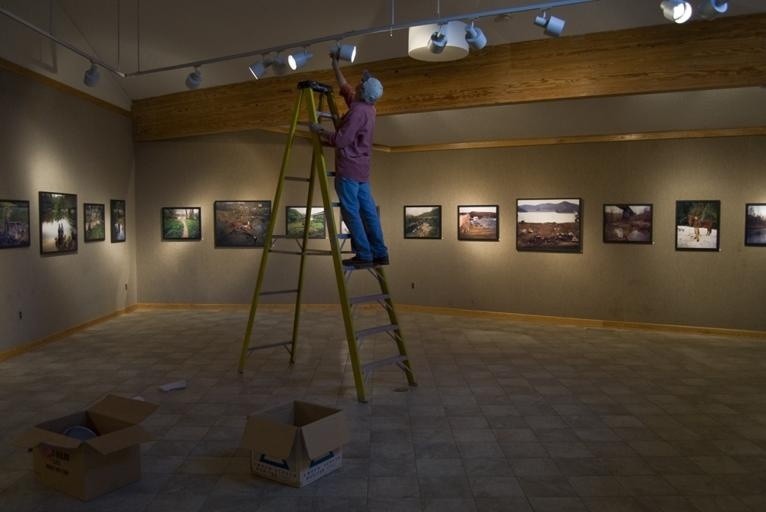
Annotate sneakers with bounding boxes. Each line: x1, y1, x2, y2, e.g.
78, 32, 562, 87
343, 257, 373, 268
374, 256, 388, 265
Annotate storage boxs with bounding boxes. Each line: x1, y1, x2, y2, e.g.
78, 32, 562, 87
240, 398, 351, 490
15, 393, 161, 501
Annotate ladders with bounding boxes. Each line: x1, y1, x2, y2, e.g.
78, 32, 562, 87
237, 80, 418, 403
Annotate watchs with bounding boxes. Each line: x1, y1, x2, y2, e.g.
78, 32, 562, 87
317, 127, 324, 136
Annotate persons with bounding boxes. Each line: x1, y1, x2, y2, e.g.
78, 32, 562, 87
310, 52, 389, 269
693, 215, 701, 242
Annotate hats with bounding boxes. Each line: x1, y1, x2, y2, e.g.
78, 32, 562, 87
361, 72, 383, 104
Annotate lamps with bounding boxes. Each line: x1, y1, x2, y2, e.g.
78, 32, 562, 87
82, 61, 100, 86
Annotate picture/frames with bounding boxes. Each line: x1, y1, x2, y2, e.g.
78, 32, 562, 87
602, 202, 653, 244
160, 206, 201, 241
0, 187, 126, 256
402, 204, 442, 240
674, 199, 720, 251
516, 198, 582, 253
284, 201, 380, 242
213, 199, 271, 248
457, 204, 500, 242
744, 201, 766, 247
